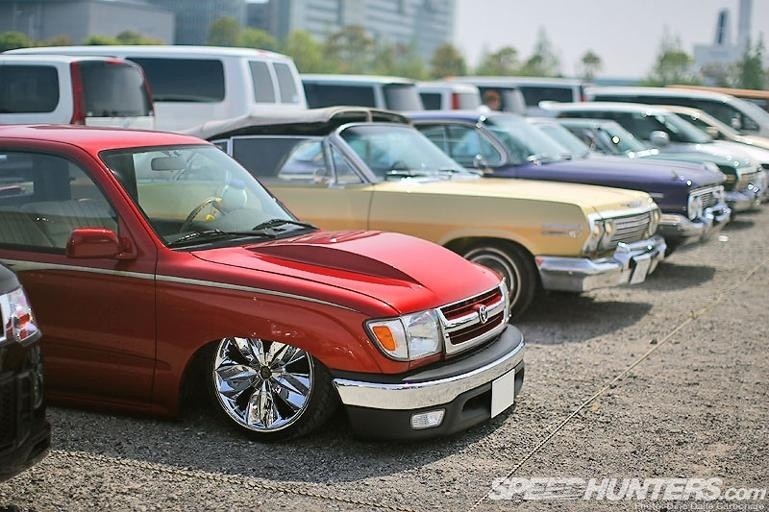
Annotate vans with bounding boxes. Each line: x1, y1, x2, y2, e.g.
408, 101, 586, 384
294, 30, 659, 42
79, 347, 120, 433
584, 85, 769, 141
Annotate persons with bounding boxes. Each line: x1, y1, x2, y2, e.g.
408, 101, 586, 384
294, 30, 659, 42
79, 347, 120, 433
468, 90, 500, 156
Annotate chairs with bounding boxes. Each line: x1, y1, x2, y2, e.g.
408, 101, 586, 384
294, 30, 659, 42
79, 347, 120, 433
0, 198, 120, 248
